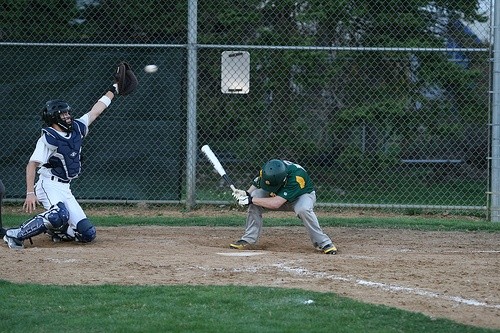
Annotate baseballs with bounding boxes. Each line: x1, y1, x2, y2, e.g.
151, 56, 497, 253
145, 65, 158, 72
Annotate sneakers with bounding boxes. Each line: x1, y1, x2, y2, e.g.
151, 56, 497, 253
3, 229, 24, 249
323, 244, 337, 255
229, 240, 255, 250
44, 228, 64, 243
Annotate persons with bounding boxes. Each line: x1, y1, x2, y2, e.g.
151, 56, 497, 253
230, 159, 337, 254
3, 61, 138, 249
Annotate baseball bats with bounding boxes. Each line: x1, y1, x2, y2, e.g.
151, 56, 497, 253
201, 145, 246, 207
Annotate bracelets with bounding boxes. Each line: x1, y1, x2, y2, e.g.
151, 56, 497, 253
27, 192, 34, 195
98, 95, 112, 107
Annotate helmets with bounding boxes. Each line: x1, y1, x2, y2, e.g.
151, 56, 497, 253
41, 99, 72, 127
260, 159, 289, 193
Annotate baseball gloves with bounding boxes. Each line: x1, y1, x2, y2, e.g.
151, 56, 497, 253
109, 62, 138, 96
